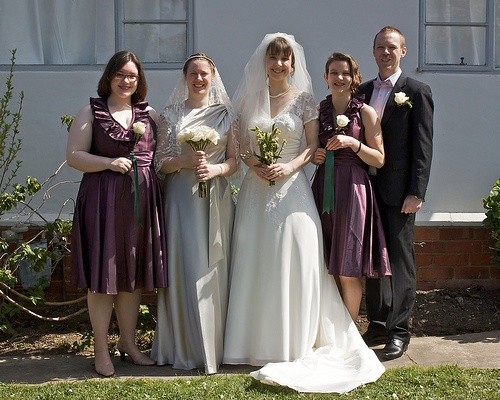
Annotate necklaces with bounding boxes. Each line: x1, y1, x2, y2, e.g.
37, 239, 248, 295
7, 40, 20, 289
270, 85, 291, 98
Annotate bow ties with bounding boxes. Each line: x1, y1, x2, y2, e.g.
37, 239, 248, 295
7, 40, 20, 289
373, 80, 392, 89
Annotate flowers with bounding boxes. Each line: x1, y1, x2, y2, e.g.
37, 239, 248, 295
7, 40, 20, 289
120, 121, 147, 226
394, 92, 414, 110
175, 124, 221, 198
237, 122, 288, 186
309, 114, 351, 215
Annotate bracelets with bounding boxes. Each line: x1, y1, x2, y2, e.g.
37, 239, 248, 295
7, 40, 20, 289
355, 141, 362, 153
218, 164, 223, 175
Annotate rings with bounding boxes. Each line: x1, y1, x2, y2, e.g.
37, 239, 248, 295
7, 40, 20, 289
202, 174, 205, 177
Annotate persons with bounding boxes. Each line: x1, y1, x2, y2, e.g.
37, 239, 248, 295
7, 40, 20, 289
352, 24, 434, 360
67, 52, 166, 376
157, 53, 239, 375
223, 32, 323, 367
310, 52, 394, 323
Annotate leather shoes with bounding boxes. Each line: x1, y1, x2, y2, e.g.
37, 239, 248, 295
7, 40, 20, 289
361, 323, 390, 347
381, 338, 408, 359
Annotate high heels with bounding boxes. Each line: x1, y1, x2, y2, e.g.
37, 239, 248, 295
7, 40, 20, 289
94, 345, 115, 376
118, 340, 156, 366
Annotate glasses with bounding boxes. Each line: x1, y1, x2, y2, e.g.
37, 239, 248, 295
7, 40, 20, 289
116, 73, 139, 82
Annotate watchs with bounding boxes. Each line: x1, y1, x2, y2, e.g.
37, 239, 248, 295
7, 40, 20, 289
412, 194, 422, 200
